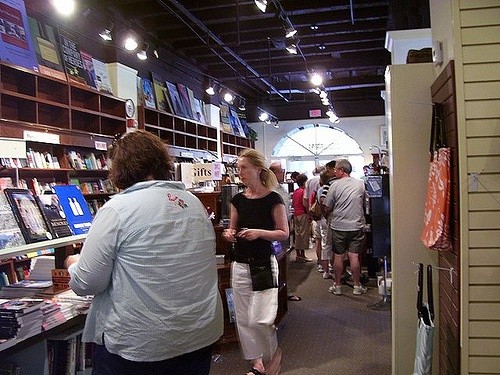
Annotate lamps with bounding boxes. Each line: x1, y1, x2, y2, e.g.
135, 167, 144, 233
98, 0, 383, 129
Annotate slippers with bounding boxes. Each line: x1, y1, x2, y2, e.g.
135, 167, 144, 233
244, 367, 267, 375
287, 294, 302, 302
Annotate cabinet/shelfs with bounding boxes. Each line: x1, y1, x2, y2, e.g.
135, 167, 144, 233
0, 62, 294, 375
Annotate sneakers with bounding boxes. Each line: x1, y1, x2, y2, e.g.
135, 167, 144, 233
329, 282, 341, 294
352, 284, 367, 295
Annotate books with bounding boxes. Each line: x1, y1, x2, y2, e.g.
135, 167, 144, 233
0, 0, 113, 94
140, 71, 209, 124
0, 146, 118, 375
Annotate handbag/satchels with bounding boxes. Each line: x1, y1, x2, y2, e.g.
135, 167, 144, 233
413, 262, 435, 375
309, 203, 322, 221
420, 101, 452, 253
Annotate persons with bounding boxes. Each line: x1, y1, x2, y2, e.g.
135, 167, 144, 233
223, 148, 289, 375
322, 158, 368, 295
64, 129, 224, 375
290, 159, 337, 279
268, 162, 302, 302
360, 165, 372, 191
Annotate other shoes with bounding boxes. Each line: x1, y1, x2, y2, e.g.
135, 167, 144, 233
296, 255, 312, 262
319, 263, 334, 272
322, 271, 333, 279
